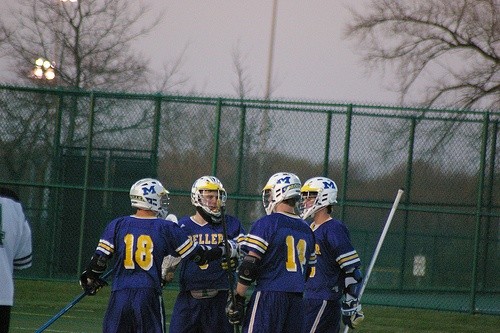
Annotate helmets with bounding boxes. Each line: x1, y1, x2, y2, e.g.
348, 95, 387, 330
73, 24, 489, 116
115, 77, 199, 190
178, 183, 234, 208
299, 176, 338, 221
129, 178, 170, 220
261, 172, 301, 216
190, 176, 227, 224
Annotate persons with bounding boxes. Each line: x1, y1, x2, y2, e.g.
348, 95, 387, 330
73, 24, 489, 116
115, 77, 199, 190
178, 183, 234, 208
0, 187, 34, 333
159, 174, 250, 333
77, 177, 244, 333
224, 171, 318, 333
298, 176, 366, 333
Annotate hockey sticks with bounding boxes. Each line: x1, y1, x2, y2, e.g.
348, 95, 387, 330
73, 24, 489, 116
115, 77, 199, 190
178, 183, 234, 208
343, 187, 405, 333
35, 269, 113, 333
217, 199, 239, 333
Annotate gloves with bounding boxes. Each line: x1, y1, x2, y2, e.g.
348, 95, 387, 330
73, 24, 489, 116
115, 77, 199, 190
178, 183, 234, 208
341, 298, 364, 330
79, 270, 108, 295
225, 295, 246, 325
220, 240, 237, 260
